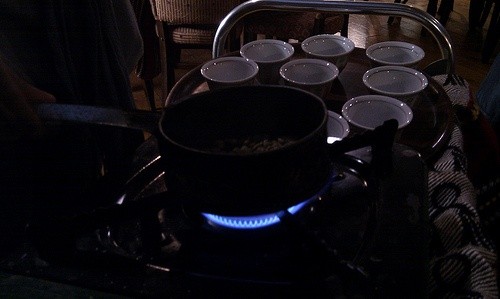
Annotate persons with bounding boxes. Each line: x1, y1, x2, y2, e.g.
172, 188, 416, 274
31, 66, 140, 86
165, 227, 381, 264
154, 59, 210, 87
0, 0, 161, 185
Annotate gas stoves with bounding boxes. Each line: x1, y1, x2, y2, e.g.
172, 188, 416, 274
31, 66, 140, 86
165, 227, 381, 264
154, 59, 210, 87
77, 101, 427, 299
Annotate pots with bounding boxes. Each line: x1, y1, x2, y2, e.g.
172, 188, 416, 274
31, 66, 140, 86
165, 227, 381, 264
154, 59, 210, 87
7, 83, 330, 202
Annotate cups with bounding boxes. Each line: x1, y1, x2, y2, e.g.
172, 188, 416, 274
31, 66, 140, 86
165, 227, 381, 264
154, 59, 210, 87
200, 57, 259, 90
325, 109, 350, 145
279, 59, 339, 100
365, 42, 426, 71
362, 66, 428, 107
240, 39, 295, 85
301, 34, 355, 74
342, 95, 413, 135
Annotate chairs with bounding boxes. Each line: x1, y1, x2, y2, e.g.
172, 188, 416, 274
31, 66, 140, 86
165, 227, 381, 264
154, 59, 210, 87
140, 0, 350, 111
